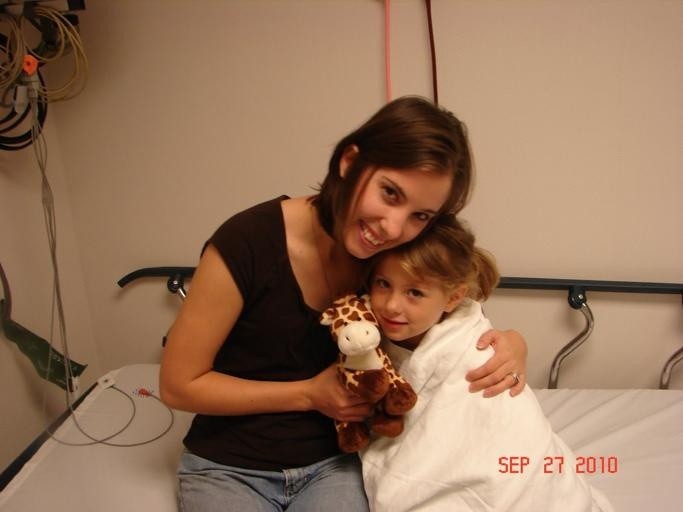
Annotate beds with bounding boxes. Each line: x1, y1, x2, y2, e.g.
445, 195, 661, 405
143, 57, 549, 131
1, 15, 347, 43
0, 268, 682, 511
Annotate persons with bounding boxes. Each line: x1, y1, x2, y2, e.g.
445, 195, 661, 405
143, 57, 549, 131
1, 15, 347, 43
160, 97, 475, 511
364, 214, 591, 511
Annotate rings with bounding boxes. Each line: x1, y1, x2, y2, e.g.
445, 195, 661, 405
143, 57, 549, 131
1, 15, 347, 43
510, 371, 522, 381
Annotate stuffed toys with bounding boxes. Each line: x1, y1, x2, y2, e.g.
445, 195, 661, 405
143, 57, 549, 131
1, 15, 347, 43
319, 294, 418, 454
